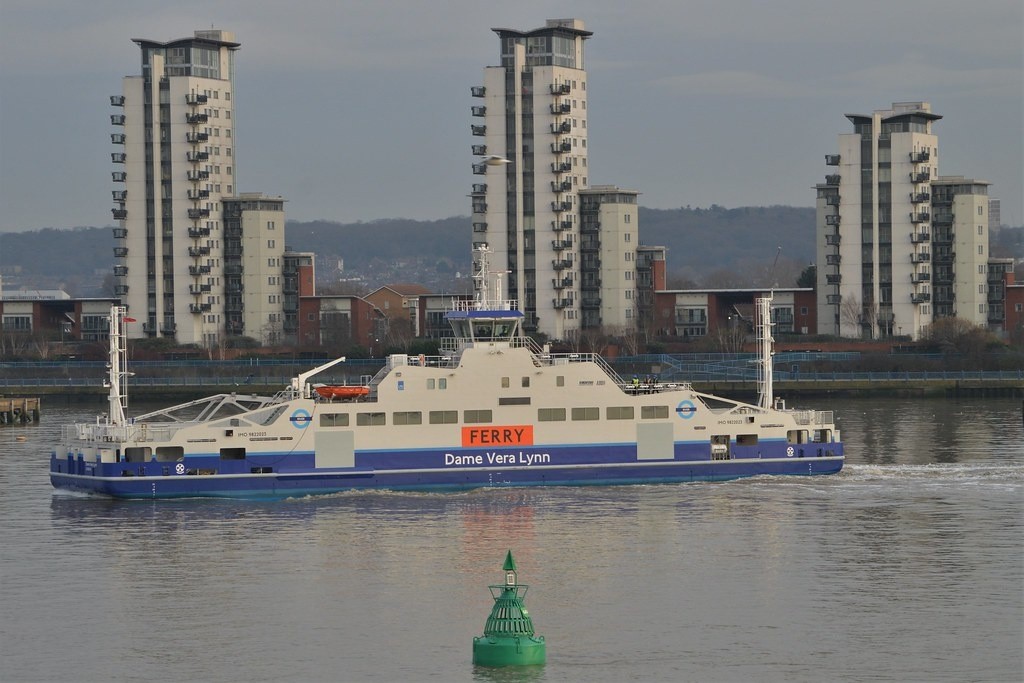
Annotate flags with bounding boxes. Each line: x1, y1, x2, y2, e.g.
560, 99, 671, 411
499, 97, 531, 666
122, 316, 136, 323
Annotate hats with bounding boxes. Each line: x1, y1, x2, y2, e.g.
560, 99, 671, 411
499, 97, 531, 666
646, 374, 649, 377
633, 374, 637, 378
653, 375, 657, 378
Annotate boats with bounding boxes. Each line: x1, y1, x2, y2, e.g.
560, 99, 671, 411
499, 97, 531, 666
315, 385, 371, 401
46, 284, 847, 506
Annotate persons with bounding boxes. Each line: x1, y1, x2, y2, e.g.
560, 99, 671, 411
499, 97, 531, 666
652, 375, 659, 394
631, 374, 640, 396
479, 326, 491, 341
643, 374, 652, 394
